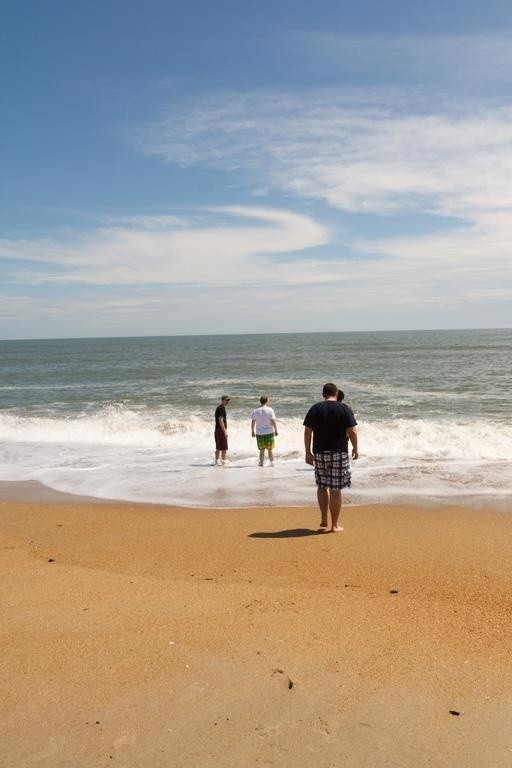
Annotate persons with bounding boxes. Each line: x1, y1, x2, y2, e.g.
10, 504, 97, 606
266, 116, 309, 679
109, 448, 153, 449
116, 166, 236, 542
307, 383, 360, 536
212, 395, 231, 466
251, 397, 279, 466
339, 391, 346, 402
